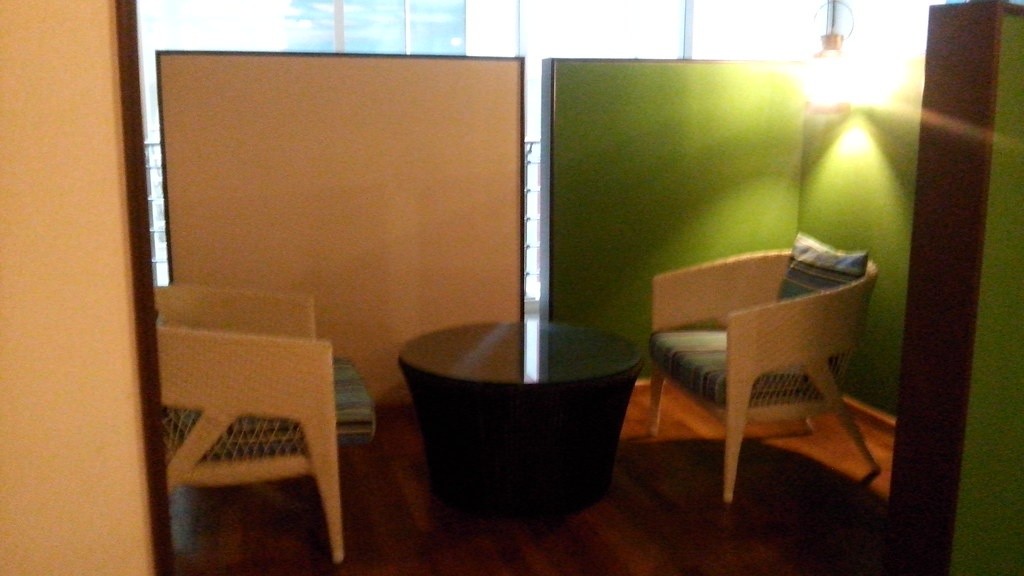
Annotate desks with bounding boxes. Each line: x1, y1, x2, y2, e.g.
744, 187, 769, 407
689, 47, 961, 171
397, 324, 644, 531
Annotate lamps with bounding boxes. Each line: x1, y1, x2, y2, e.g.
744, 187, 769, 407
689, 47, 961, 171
792, 0, 872, 108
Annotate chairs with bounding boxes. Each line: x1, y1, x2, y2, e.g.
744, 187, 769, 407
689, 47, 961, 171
646, 251, 882, 508
154, 284, 345, 564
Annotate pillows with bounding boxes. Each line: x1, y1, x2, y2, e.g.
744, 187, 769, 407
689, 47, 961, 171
777, 231, 870, 301
